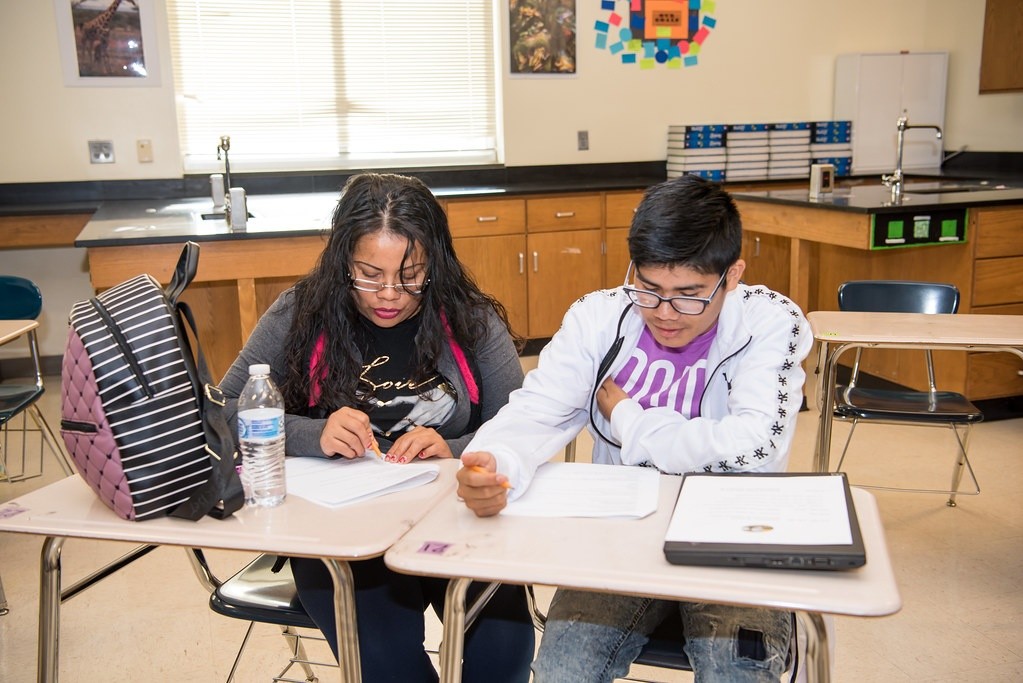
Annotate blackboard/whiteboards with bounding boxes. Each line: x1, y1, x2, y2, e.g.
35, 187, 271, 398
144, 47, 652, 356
833, 51, 948, 175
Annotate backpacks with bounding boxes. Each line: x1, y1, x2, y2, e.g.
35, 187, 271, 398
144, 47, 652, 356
58, 240, 243, 522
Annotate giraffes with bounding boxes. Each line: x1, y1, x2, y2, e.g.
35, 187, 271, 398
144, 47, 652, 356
79, 0, 136, 68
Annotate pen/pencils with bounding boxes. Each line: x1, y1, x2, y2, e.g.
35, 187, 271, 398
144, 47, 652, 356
371, 443, 384, 462
472, 465, 515, 491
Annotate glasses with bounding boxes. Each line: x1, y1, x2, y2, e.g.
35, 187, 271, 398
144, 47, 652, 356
348, 273, 431, 293
622, 260, 729, 315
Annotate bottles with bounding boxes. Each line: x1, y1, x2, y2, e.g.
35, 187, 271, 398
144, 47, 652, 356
237, 364, 287, 509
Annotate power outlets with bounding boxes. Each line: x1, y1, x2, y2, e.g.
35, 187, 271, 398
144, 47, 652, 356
88, 141, 114, 163
578, 131, 588, 149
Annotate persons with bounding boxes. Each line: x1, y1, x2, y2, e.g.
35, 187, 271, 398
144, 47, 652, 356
455, 174, 813, 683
216, 174, 535, 683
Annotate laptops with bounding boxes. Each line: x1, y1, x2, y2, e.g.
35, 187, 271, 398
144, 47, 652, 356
663, 472, 867, 571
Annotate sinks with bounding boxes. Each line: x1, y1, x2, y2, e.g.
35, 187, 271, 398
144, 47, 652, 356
903, 186, 1009, 195
201, 211, 256, 220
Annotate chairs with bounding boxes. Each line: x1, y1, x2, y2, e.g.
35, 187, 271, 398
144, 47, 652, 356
0, 272, 75, 482
832, 283, 984, 508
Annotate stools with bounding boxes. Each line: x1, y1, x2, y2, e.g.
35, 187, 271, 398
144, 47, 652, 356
185, 542, 441, 683
618, 599, 829, 683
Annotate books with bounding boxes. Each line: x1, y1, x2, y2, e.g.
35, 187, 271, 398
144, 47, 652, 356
666, 121, 853, 182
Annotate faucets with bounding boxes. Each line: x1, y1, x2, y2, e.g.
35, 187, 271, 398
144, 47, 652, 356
216, 135, 231, 219
881, 117, 943, 193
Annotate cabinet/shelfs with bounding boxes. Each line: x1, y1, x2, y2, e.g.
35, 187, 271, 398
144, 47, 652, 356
435, 190, 1023, 419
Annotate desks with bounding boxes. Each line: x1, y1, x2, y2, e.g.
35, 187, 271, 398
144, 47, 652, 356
808, 310, 1022, 465
384, 463, 904, 683
74, 195, 337, 346
1, 320, 39, 344
0, 450, 466, 682
